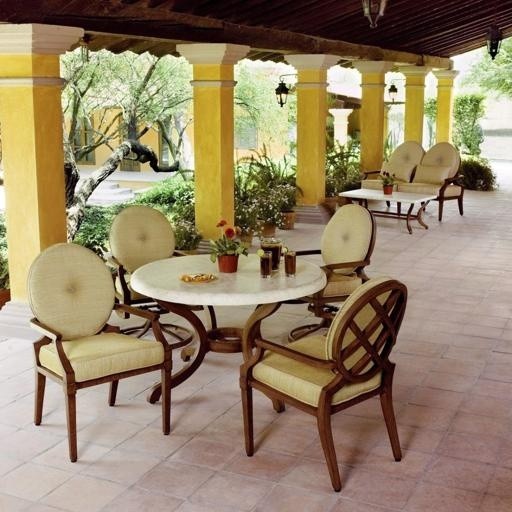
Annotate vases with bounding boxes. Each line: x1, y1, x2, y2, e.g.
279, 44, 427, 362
218, 256, 238, 272
240, 233, 252, 247
384, 185, 393, 194
328, 197, 336, 209
176, 250, 198, 254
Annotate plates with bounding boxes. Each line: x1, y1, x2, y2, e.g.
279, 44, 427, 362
181, 273, 218, 285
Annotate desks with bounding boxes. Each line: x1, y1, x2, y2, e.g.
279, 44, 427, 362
339, 189, 437, 234
130, 254, 327, 412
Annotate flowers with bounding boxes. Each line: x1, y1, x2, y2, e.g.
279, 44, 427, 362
326, 176, 337, 196
210, 221, 248, 263
174, 218, 202, 250
234, 184, 296, 241
378, 171, 407, 186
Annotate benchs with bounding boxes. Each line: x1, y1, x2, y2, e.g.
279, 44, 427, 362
363, 141, 465, 221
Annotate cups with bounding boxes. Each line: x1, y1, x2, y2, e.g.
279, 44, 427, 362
259, 238, 296, 278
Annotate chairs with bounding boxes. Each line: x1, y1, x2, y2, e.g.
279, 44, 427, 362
27, 243, 171, 462
240, 277, 407, 491
109, 206, 204, 349
281, 204, 376, 343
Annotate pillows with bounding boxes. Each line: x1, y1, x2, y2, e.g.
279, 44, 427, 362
412, 164, 452, 185
379, 162, 417, 182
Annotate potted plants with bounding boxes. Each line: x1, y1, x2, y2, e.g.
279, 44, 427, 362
337, 180, 355, 207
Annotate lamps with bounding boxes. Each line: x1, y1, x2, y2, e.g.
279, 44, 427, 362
388, 78, 405, 104
274, 73, 297, 108
360, 0, 388, 30
484, 24, 504, 61
